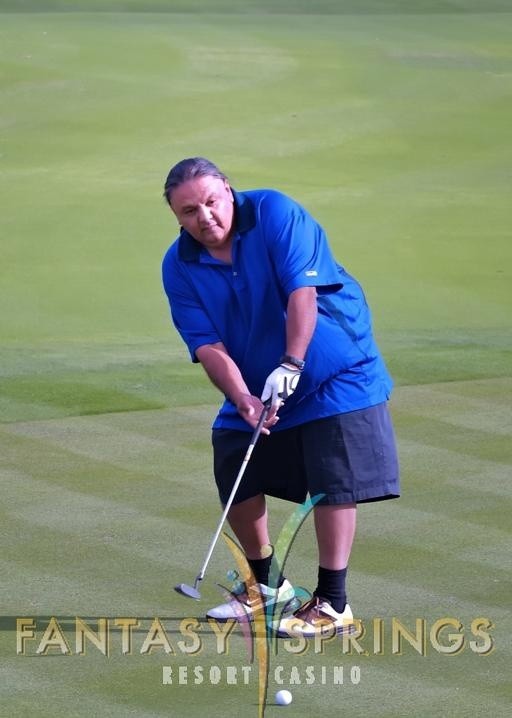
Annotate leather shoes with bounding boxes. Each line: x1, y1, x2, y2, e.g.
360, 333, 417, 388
207, 579, 299, 620
268, 599, 355, 637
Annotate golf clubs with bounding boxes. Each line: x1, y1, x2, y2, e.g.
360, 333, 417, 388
174, 401, 271, 598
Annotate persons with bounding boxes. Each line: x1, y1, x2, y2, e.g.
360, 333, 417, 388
162, 156, 403, 638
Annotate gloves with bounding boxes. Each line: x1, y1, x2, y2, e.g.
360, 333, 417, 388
261, 364, 301, 414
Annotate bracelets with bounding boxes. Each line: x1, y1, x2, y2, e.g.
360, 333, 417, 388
280, 354, 306, 370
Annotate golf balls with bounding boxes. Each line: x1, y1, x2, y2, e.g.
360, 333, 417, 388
275, 690, 292, 705
